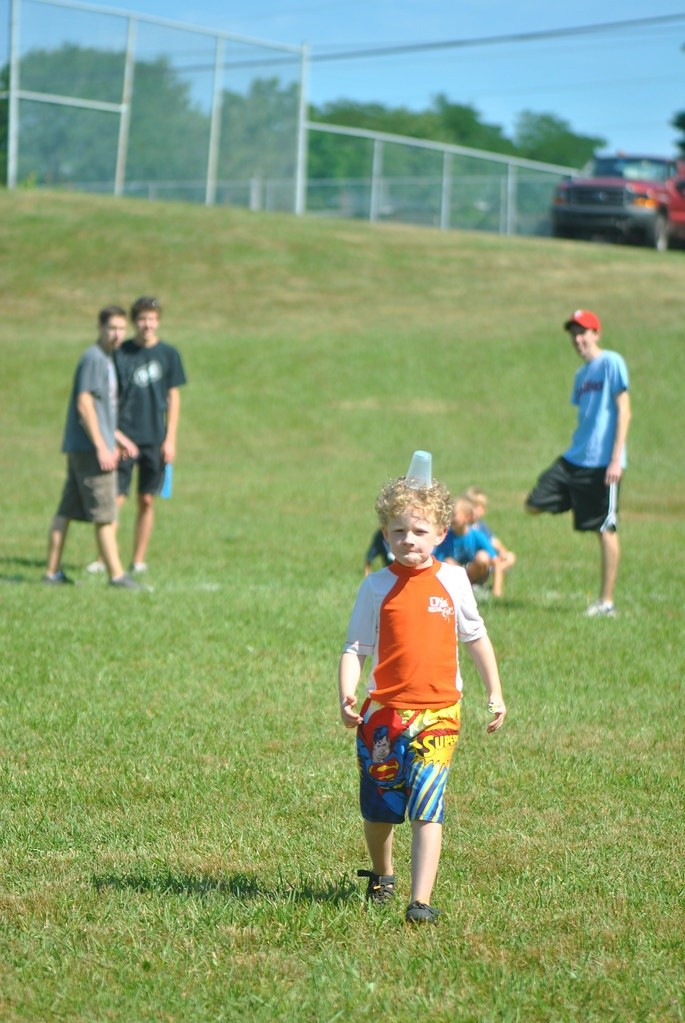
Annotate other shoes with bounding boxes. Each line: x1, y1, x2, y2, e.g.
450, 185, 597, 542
357, 870, 395, 904
40, 569, 75, 585
582, 599, 616, 617
87, 561, 106, 572
130, 561, 146, 572
110, 571, 139, 587
406, 900, 440, 923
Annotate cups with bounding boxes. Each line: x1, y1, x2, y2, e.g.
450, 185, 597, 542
404, 450, 433, 492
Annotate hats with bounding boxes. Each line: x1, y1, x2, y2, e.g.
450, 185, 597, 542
564, 309, 600, 331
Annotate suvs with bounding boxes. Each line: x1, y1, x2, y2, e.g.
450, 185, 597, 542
552, 139, 685, 252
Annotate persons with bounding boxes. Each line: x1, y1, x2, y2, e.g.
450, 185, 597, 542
44, 307, 141, 585
364, 486, 515, 602
87, 296, 185, 573
338, 474, 510, 925
526, 310, 631, 615
